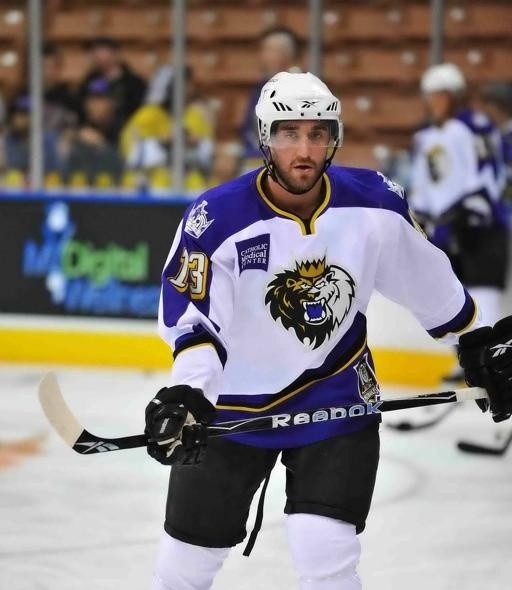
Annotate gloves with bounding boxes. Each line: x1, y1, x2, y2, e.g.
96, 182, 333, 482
456, 315, 512, 422
144, 384, 218, 466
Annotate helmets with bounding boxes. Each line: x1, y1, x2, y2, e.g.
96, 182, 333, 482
420, 62, 467, 97
254, 69, 345, 148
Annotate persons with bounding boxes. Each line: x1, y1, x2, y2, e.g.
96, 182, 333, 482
144, 66, 512, 588
403, 60, 512, 385
481, 79, 511, 169
237, 27, 309, 174
1, 23, 214, 193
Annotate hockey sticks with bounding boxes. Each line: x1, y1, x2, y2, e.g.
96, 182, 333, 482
37, 372, 487, 455
